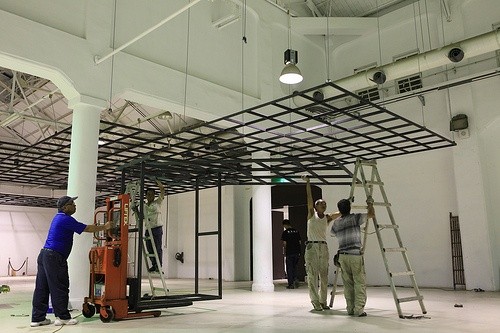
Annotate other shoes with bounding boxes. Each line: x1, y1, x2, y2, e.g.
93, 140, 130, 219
156, 266, 164, 274
321, 303, 330, 310
348, 310, 354, 315
312, 300, 323, 311
295, 285, 300, 288
148, 266, 155, 272
286, 285, 294, 289
359, 312, 367, 317
28, 318, 51, 327
54, 317, 77, 326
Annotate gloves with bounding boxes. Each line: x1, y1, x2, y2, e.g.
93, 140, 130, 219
301, 174, 310, 181
365, 195, 375, 204
347, 196, 354, 202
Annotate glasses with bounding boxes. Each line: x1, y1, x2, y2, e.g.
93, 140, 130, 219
65, 204, 76, 207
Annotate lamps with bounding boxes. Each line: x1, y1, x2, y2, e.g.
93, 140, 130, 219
279, 14, 303, 84
158, 111, 172, 120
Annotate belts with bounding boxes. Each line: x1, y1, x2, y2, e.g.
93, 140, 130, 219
146, 226, 162, 231
339, 251, 364, 255
41, 248, 60, 255
305, 240, 328, 245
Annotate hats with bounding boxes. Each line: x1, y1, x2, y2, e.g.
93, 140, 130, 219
57, 196, 79, 206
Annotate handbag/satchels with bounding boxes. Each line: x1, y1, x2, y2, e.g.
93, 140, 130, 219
333, 250, 341, 268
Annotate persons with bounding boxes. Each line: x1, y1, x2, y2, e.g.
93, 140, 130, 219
279, 220, 301, 290
301, 174, 355, 311
30, 196, 116, 326
331, 196, 375, 317
143, 178, 165, 275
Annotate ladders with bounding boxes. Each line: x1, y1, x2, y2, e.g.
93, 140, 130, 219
119, 184, 169, 295
329, 158, 427, 320
